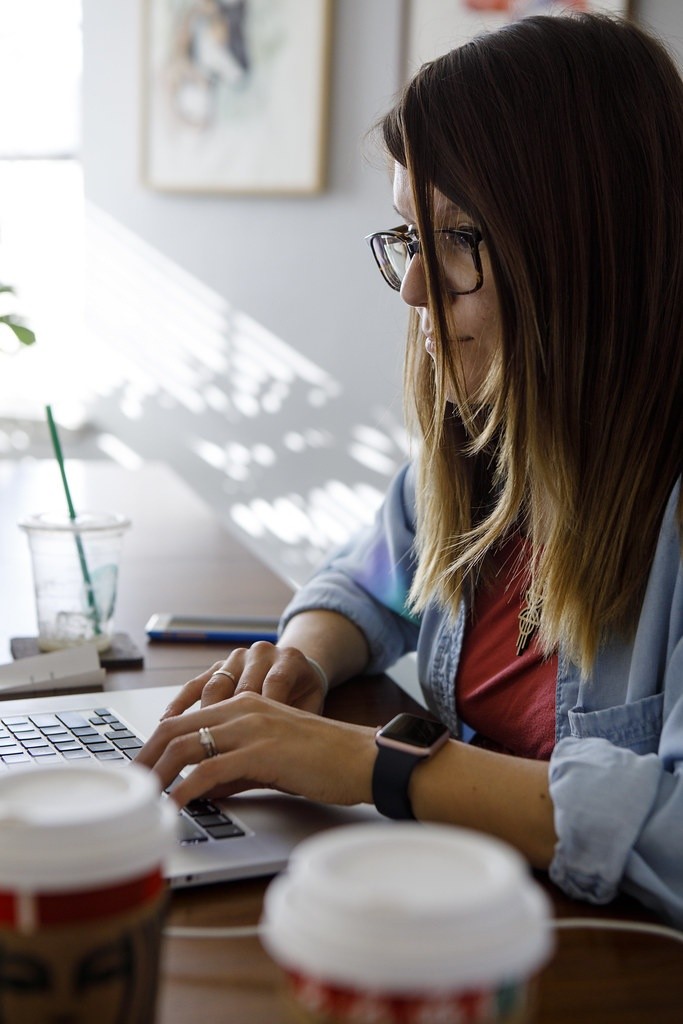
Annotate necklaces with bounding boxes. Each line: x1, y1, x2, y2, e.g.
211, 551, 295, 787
510, 519, 548, 657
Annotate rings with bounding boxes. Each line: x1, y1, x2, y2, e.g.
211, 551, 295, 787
212, 670, 237, 687
200, 724, 218, 759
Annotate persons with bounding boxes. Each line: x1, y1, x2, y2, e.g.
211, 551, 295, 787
132, 10, 682, 928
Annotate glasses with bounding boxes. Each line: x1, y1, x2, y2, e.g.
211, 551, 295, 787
365, 224, 484, 295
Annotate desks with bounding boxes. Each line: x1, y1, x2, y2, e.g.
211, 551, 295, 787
0, 628, 682, 1024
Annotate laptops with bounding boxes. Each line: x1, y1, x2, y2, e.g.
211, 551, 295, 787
0, 682, 390, 891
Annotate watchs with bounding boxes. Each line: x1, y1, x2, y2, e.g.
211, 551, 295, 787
373, 712, 452, 822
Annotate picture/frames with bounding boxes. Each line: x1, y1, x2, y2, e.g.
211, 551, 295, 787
142, 0, 337, 196
396, 1, 630, 95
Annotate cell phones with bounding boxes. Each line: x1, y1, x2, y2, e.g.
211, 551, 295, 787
144, 614, 281, 644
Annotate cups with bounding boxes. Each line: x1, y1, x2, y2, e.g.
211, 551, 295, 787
21, 521, 133, 650
258, 824, 555, 1024
3, 764, 164, 1021
